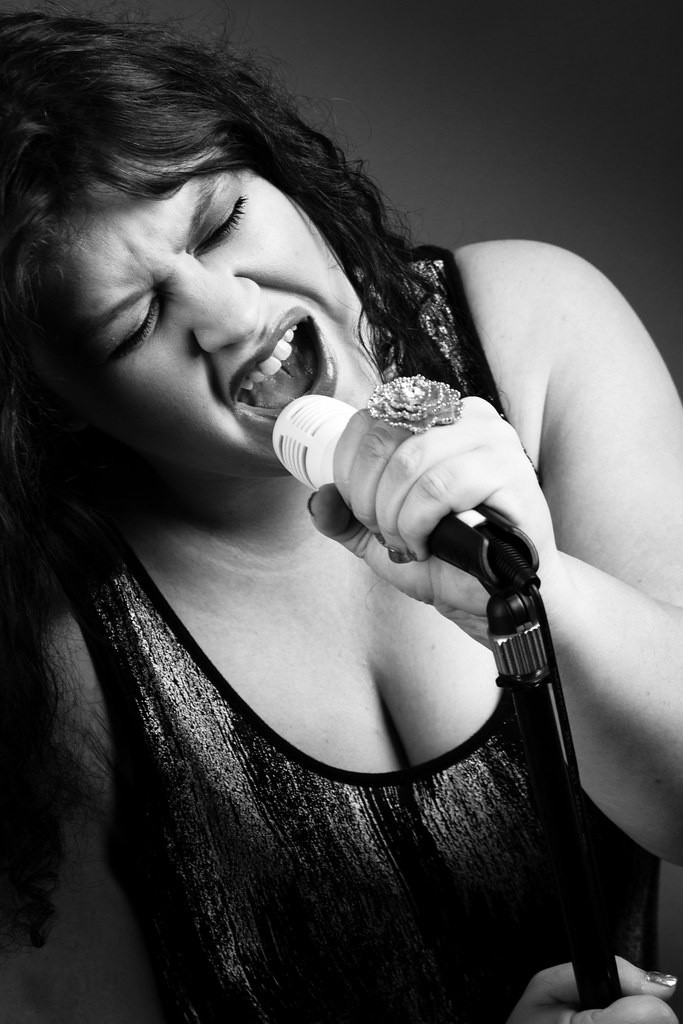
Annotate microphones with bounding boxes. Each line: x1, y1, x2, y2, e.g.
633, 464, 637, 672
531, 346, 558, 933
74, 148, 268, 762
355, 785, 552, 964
271, 392, 541, 598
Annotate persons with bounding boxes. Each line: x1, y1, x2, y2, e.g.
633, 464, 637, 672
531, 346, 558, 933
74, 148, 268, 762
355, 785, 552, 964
0, 11, 683, 1024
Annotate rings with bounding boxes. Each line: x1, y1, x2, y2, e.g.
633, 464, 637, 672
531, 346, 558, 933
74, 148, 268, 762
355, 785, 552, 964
368, 374, 465, 435
